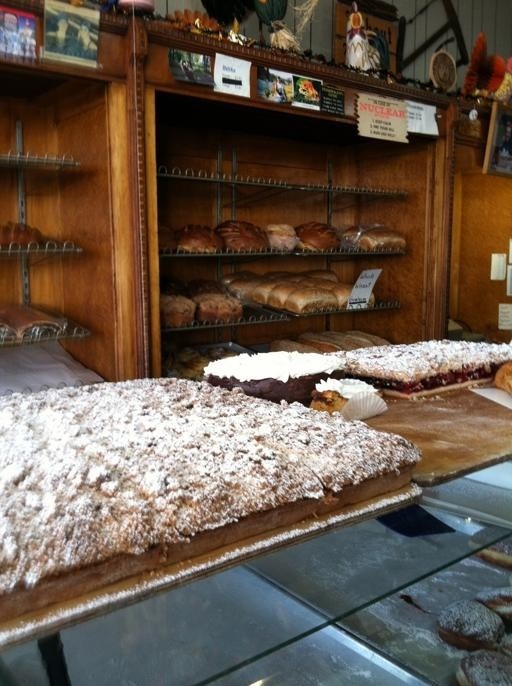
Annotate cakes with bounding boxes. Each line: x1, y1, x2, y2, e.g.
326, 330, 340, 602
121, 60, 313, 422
202, 348, 347, 408
324, 337, 512, 394
0, 377, 423, 627
493, 359, 512, 395
1, 302, 63, 338
308, 376, 383, 417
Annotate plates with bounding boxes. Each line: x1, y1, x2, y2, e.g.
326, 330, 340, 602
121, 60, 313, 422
429, 52, 457, 92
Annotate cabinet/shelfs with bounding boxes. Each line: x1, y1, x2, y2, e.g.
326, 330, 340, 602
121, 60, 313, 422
442, 86, 512, 341
0, 0, 136, 388
134, 13, 452, 378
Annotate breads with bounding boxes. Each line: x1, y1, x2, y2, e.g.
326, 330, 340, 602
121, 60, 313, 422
0, 220, 45, 248
269, 328, 391, 353
155, 225, 177, 254
159, 274, 180, 296
191, 292, 243, 325
213, 219, 269, 253
160, 296, 197, 327
265, 222, 298, 252
340, 224, 408, 253
469, 526, 512, 568
185, 276, 230, 299
167, 345, 208, 382
207, 342, 239, 360
295, 220, 340, 253
220, 268, 376, 315
436, 585, 512, 685
175, 223, 221, 253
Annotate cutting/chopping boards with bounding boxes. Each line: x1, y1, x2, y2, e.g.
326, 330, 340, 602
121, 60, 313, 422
338, 395, 512, 490
0, 463, 411, 621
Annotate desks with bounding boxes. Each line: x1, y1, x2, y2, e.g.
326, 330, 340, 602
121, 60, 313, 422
256, 368, 512, 487
0, 482, 425, 686
